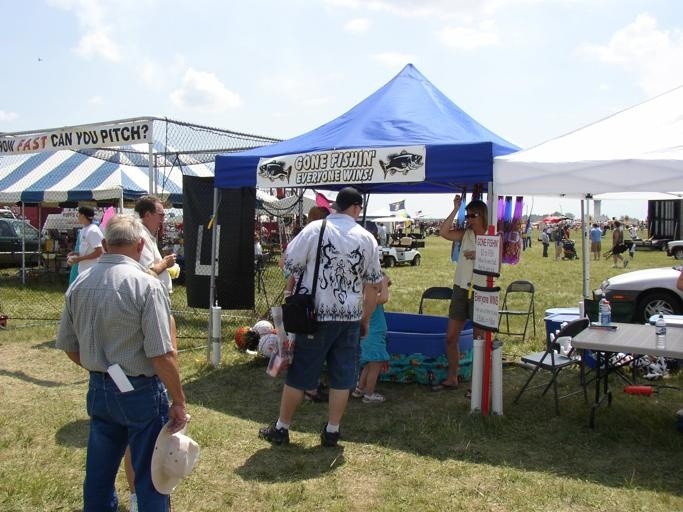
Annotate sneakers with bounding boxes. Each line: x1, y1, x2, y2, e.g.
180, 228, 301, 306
130, 493, 138, 512
260, 419, 288, 445
320, 422, 340, 447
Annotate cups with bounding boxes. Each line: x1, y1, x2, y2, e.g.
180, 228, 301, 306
579, 301, 584, 315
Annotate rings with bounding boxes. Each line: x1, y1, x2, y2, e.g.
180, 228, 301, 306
470, 256, 472, 259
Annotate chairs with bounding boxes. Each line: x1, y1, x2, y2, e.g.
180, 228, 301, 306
497, 279, 537, 341
514, 319, 588, 416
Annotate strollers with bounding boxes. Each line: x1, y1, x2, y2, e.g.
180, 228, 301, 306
562, 237, 576, 261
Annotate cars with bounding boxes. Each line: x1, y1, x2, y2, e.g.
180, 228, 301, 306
666, 239, 683, 260
0, 208, 47, 265
584, 264, 683, 326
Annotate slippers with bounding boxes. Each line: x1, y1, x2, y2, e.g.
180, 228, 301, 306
430, 381, 458, 392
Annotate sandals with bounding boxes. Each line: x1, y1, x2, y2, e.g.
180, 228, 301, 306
352, 386, 366, 398
304, 382, 329, 403
362, 393, 386, 404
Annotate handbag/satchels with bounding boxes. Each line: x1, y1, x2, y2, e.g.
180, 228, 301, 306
282, 287, 316, 334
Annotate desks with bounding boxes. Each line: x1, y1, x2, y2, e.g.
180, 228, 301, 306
571, 321, 682, 430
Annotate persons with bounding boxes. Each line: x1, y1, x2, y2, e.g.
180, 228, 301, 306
65, 204, 107, 275
278, 206, 328, 403
520, 215, 649, 269
374, 209, 426, 251
130, 192, 178, 299
675, 270, 682, 419
254, 185, 385, 448
54, 212, 189, 511
122, 441, 138, 508
349, 274, 390, 404
429, 194, 489, 398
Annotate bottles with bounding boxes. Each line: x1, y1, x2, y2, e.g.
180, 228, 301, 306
597, 292, 611, 326
654, 315, 666, 350
623, 385, 653, 397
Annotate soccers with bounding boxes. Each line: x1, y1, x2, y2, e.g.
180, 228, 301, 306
234, 319, 278, 354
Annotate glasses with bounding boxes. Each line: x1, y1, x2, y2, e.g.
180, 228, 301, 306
465, 213, 479, 218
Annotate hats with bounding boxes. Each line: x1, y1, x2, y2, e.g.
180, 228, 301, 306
329, 187, 362, 212
79, 207, 94, 220
151, 414, 200, 495
